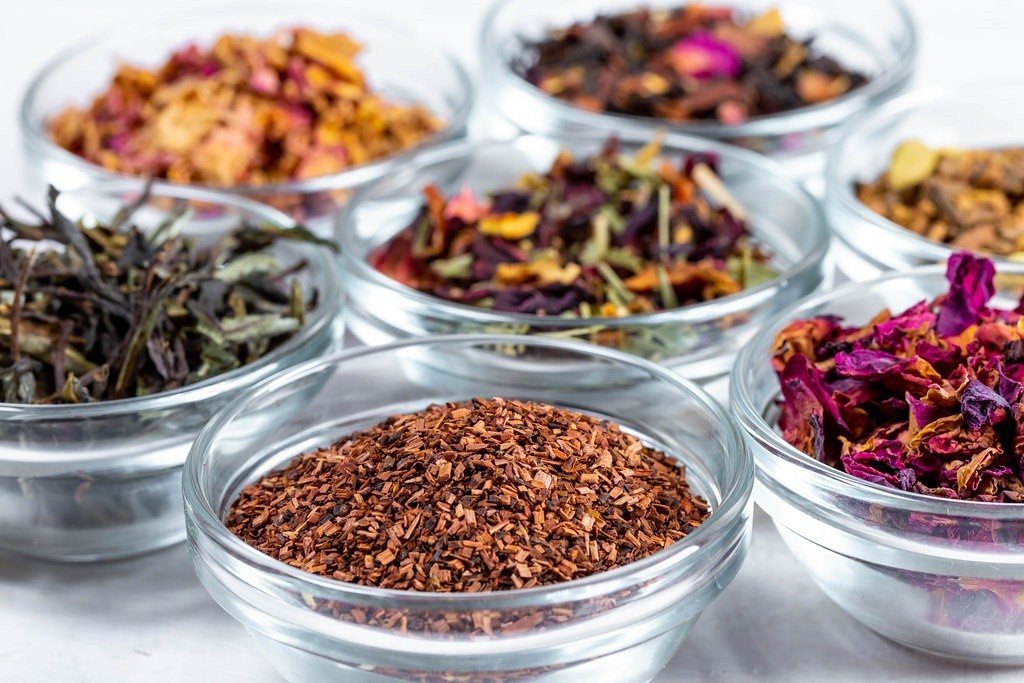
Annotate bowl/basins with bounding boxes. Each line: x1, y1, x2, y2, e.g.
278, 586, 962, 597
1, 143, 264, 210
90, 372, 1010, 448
0, 176, 343, 564
331, 124, 829, 411
181, 333, 756, 683
730, 261, 1024, 670
16, 0, 1024, 290
